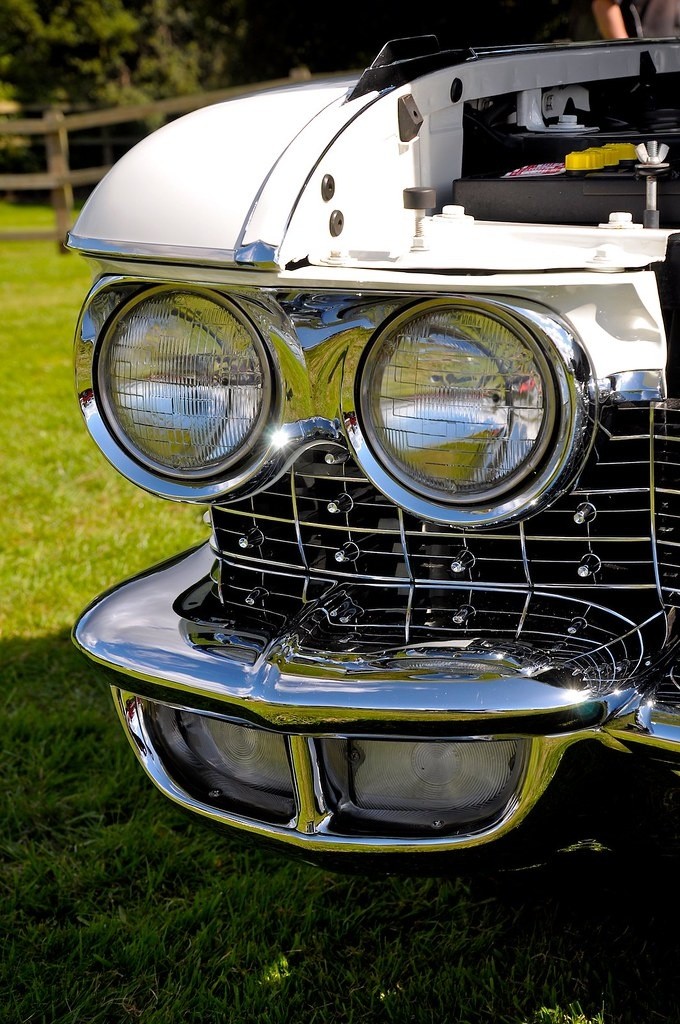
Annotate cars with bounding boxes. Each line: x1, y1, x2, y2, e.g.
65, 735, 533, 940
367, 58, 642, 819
65, 0, 680, 873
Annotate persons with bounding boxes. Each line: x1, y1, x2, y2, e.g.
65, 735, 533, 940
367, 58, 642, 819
591, 0, 680, 37
